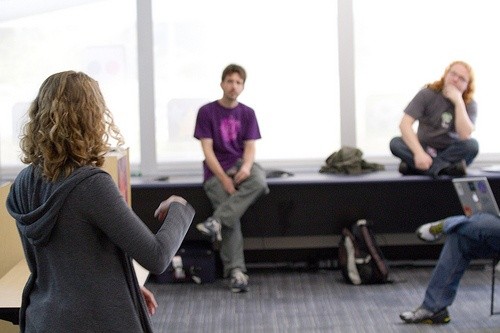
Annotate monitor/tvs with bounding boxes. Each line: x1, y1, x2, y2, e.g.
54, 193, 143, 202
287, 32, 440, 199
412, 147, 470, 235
451, 177, 500, 219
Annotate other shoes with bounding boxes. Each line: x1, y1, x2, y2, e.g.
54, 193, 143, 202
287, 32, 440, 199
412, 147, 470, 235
400, 161, 412, 175
455, 159, 466, 176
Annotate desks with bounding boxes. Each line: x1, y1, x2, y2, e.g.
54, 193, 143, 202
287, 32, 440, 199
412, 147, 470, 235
131, 167, 500, 272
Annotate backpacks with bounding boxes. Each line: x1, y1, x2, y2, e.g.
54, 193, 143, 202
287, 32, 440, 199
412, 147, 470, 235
338, 220, 389, 286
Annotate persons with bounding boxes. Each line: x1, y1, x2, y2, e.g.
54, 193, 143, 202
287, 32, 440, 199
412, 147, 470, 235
400, 213, 500, 324
390, 61, 479, 178
193, 64, 266, 292
5, 71, 195, 333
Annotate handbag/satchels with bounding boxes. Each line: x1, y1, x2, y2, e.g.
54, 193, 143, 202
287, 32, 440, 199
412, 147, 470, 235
156, 248, 215, 284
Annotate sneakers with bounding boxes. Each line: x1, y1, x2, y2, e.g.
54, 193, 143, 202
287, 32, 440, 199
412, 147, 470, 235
399, 302, 450, 325
417, 221, 444, 243
196, 217, 222, 241
229, 268, 249, 293
